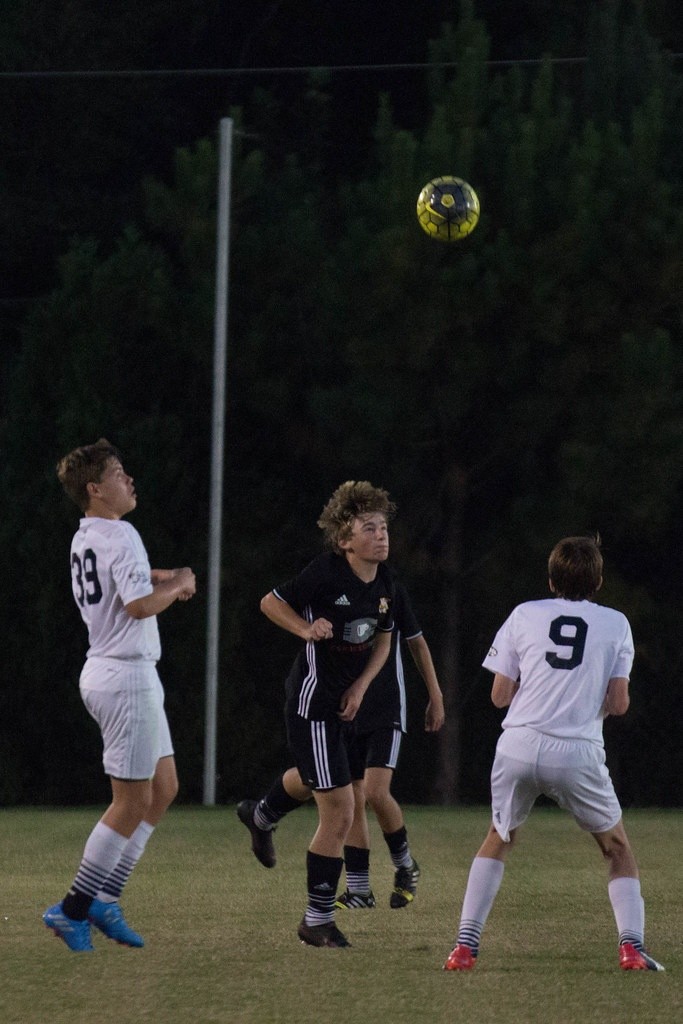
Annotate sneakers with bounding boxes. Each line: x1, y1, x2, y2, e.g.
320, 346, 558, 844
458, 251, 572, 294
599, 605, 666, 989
618, 942, 666, 971
442, 944, 477, 970
298, 915, 353, 948
390, 858, 420, 908
89, 896, 144, 947
237, 801, 277, 868
334, 887, 375, 909
42, 900, 96, 952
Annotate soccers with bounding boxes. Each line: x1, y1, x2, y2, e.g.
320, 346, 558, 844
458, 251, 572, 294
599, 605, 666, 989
416, 176, 480, 242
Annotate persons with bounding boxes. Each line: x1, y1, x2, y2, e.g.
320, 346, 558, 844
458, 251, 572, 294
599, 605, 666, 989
442, 537, 667, 971
336, 566, 446, 910
43, 439, 196, 952
238, 481, 396, 948
